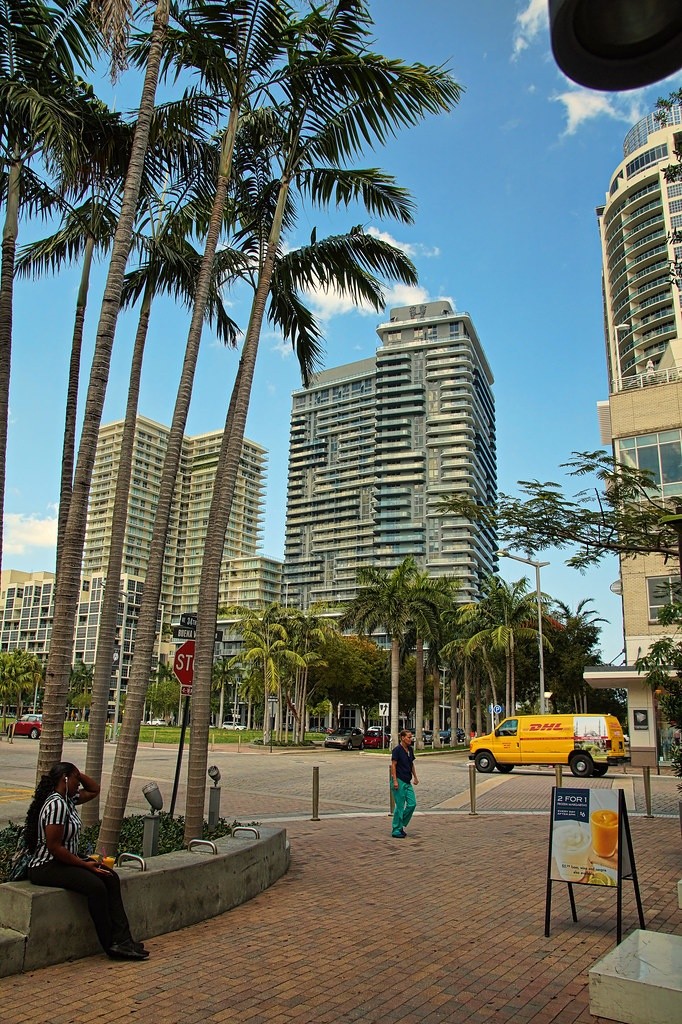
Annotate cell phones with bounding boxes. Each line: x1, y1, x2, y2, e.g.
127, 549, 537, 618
98, 868, 111, 873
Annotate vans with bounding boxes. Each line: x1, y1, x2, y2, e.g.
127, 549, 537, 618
468, 714, 627, 777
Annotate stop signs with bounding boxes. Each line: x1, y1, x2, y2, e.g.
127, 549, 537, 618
172, 640, 196, 686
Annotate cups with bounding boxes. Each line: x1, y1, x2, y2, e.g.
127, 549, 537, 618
103, 856, 116, 869
591, 809, 619, 857
87, 853, 100, 861
553, 826, 591, 882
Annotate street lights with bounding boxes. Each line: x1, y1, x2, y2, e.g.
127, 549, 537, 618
495, 549, 550, 714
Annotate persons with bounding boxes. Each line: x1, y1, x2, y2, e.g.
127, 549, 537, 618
24, 762, 150, 958
674, 728, 681, 755
504, 722, 517, 736
391, 730, 419, 838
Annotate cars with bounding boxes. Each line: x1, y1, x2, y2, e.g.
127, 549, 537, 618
323, 727, 364, 751
278, 722, 335, 735
363, 725, 465, 749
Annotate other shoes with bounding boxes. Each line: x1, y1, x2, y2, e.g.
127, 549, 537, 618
106, 938, 151, 961
392, 833, 405, 838
400, 830, 407, 836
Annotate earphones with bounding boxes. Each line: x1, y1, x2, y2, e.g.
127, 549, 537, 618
65, 777, 68, 782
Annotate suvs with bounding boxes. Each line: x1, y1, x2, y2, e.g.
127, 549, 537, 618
147, 718, 166, 727
6, 716, 41, 739
221, 721, 247, 731
